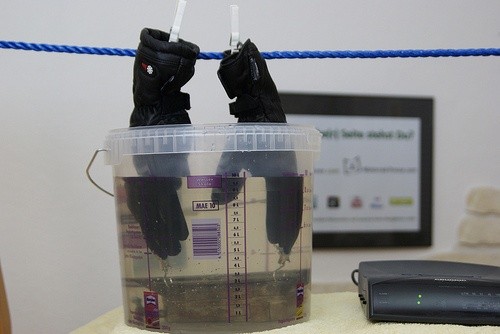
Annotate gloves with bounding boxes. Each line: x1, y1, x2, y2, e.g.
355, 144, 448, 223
210, 39, 304, 253
123, 29, 201, 260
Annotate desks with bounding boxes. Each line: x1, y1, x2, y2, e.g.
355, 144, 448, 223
61, 282, 500, 334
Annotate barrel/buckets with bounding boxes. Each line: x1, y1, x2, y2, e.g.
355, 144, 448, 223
86, 117, 321, 333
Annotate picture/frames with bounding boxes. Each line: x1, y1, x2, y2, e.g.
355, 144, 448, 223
277, 91, 433, 250
